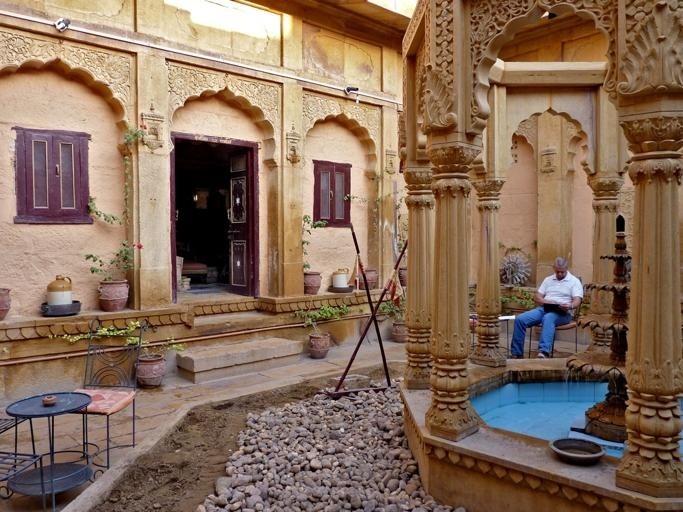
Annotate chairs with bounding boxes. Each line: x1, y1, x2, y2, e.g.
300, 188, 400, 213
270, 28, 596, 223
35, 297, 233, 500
528, 276, 583, 359
69, 318, 147, 469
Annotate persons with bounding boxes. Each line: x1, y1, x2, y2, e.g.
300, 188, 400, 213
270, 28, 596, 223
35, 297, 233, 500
509, 257, 583, 359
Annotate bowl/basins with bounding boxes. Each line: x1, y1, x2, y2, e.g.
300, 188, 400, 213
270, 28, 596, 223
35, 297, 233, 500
327, 285, 354, 294
549, 436, 605, 466
40, 300, 82, 316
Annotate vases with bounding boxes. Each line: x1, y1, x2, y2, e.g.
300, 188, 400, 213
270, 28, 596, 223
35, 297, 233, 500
359, 269, 377, 290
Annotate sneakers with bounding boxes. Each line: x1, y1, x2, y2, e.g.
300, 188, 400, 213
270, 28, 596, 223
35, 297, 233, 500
537, 353, 545, 358
508, 354, 524, 359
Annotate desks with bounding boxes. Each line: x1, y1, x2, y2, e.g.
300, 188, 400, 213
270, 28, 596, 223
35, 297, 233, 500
0, 390, 103, 512
468, 314, 510, 360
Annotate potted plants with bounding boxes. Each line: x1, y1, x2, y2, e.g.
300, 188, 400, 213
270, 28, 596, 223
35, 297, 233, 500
379, 300, 408, 342
124, 333, 188, 390
295, 302, 348, 358
84, 240, 135, 312
301, 214, 324, 294
